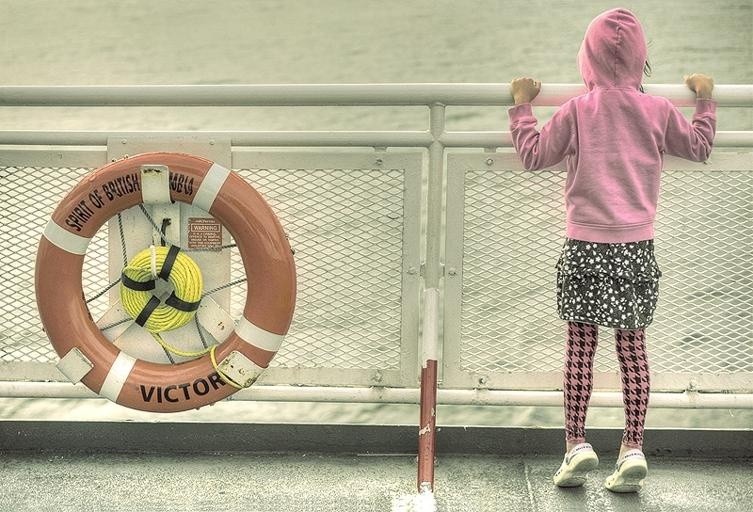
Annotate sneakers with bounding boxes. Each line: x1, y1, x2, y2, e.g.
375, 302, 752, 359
605, 450, 649, 493
551, 442, 601, 487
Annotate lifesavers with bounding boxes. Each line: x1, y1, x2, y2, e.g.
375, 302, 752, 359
34, 151, 297, 412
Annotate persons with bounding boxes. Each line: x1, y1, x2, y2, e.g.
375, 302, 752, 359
506, 6, 718, 494
